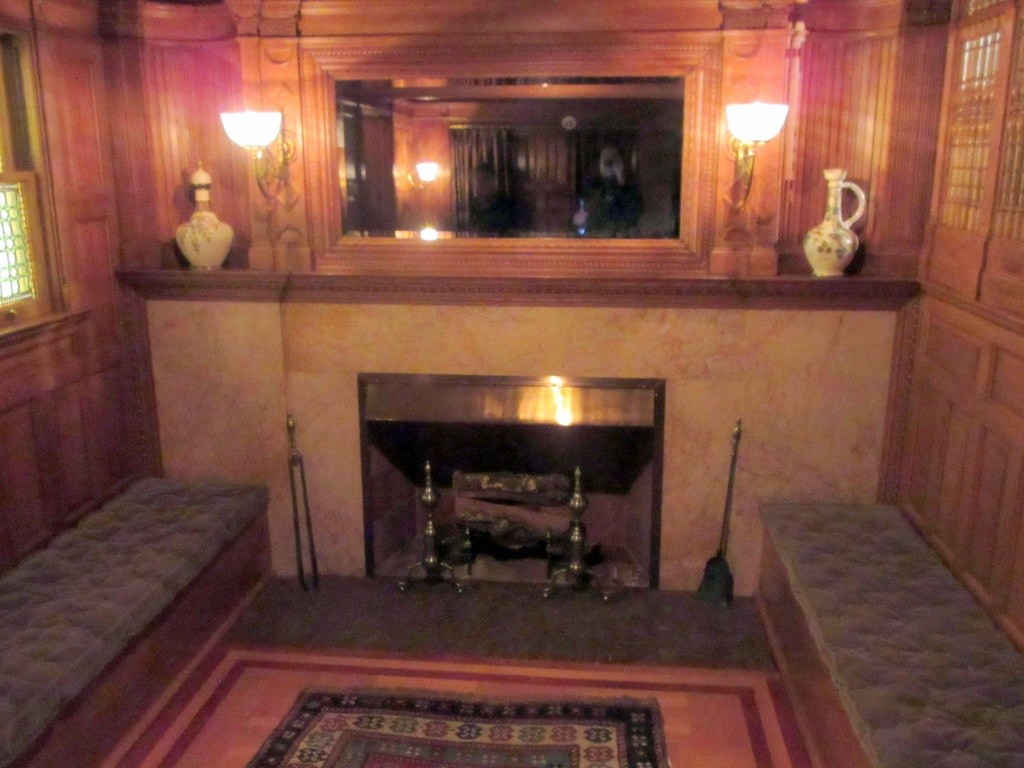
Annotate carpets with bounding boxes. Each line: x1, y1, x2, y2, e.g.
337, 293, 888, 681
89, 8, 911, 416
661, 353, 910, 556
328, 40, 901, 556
247, 685, 671, 768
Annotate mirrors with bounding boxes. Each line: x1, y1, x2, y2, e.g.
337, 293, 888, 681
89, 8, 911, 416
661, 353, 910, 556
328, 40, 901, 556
298, 36, 718, 276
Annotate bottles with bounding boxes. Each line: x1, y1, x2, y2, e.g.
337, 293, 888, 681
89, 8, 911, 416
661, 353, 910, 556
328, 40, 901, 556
803, 168, 866, 277
175, 161, 234, 271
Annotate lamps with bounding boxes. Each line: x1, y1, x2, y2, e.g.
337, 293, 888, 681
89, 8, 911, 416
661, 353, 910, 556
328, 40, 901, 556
722, 99, 790, 209
219, 108, 294, 201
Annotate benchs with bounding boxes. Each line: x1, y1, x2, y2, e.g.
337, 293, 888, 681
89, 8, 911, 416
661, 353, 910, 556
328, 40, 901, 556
0, 477, 271, 768
759, 498, 1024, 767
